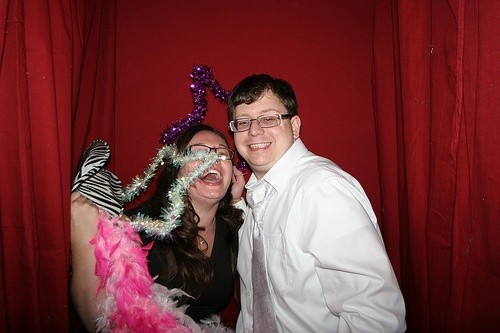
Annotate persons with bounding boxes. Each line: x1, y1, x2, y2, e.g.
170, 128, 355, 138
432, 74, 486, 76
70, 124, 244, 332
228, 73, 408, 332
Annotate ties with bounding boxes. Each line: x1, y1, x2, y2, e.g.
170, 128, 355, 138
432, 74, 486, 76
252, 188, 277, 333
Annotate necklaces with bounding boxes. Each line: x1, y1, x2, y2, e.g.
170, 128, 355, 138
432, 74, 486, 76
195, 227, 210, 244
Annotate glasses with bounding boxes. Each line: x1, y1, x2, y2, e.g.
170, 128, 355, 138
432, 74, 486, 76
182, 143, 234, 161
228, 113, 293, 133
